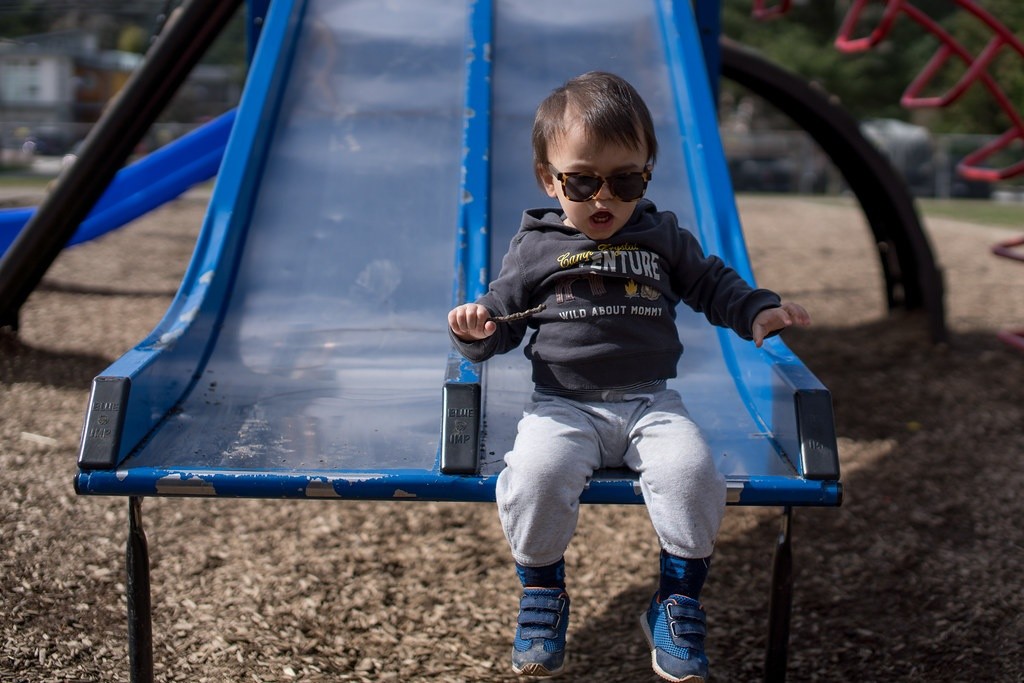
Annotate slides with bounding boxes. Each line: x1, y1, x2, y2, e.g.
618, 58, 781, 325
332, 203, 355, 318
71, 0, 847, 509
0, 105, 238, 259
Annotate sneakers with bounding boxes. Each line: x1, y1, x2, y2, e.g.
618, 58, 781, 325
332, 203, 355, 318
511, 587, 570, 676
640, 589, 710, 683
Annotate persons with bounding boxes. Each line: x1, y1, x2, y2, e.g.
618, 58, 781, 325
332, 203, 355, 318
448, 70, 811, 683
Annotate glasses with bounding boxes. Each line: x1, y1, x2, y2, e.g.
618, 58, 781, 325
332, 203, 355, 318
547, 162, 652, 203
